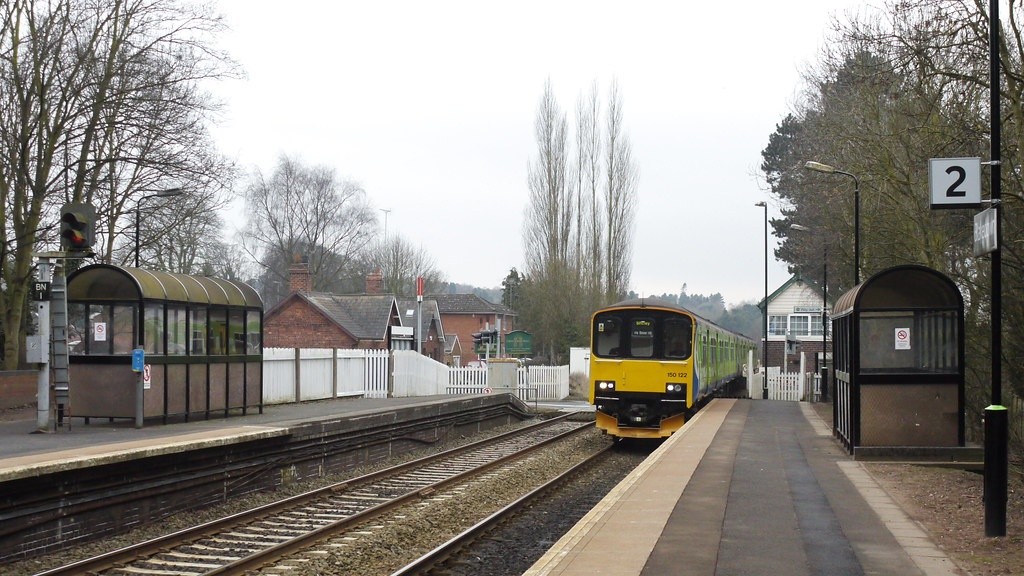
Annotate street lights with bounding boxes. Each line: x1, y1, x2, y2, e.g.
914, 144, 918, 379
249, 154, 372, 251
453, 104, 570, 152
135, 188, 183, 269
789, 223, 828, 403
804, 161, 859, 285
754, 200, 768, 399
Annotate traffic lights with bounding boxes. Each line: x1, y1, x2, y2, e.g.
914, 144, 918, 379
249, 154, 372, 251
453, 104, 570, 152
60, 202, 97, 250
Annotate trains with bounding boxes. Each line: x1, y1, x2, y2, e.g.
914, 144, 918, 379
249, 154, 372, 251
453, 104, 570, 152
588, 296, 756, 443
